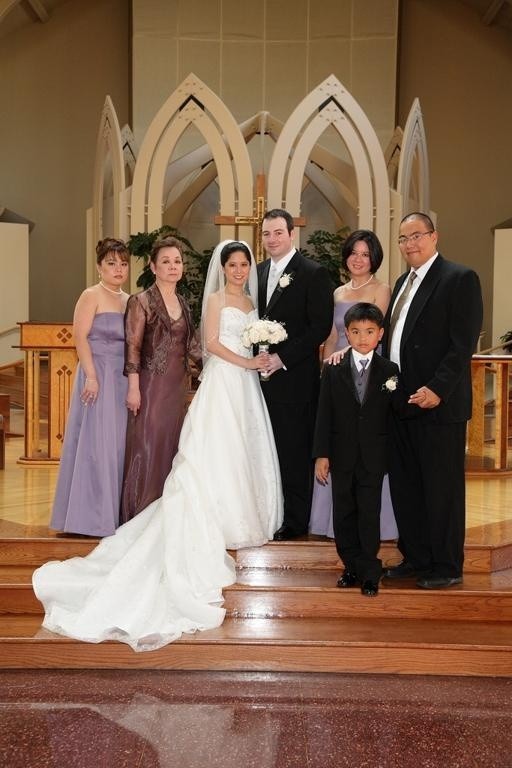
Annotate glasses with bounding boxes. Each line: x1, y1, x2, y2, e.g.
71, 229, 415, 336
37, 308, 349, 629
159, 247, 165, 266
398, 231, 433, 243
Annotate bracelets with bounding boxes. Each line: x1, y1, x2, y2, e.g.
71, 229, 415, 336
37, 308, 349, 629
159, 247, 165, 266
85, 378, 97, 382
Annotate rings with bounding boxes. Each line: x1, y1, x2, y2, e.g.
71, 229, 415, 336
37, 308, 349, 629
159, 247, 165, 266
89, 395, 93, 399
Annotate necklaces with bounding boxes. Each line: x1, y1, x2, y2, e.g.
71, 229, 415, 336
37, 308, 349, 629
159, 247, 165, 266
100, 281, 123, 294
350, 276, 374, 291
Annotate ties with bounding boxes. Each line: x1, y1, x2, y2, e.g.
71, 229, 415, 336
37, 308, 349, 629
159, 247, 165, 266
387, 271, 417, 359
265, 266, 280, 306
354, 360, 368, 384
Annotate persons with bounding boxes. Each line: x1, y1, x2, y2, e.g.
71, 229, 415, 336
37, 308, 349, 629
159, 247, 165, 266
328, 212, 483, 587
49, 238, 128, 536
31, 239, 284, 654
246, 208, 336, 540
306, 227, 400, 540
124, 236, 202, 527
313, 302, 426, 597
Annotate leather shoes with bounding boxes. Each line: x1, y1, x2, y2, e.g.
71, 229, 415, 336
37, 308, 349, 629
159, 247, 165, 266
361, 578, 380, 598
336, 571, 358, 588
384, 560, 415, 579
415, 575, 464, 590
